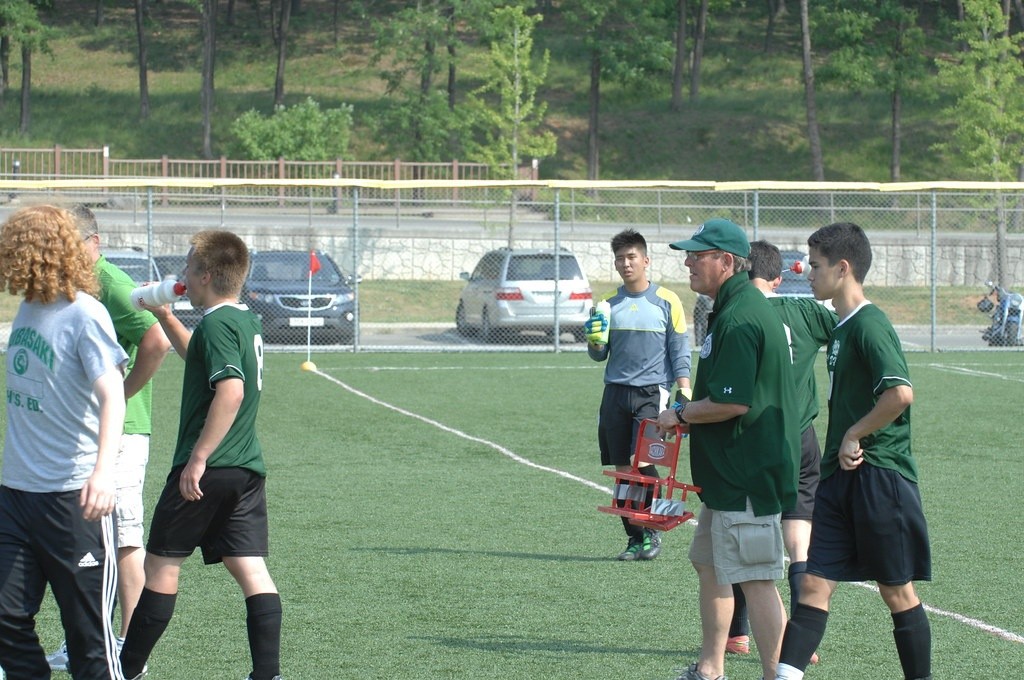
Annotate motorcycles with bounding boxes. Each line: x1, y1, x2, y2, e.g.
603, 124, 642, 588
978, 281, 1024, 346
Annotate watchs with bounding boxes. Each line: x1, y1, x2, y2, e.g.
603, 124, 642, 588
675, 402, 687, 424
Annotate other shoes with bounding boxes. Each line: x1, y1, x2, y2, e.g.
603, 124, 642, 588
46, 640, 69, 672
674, 662, 725, 680
725, 635, 750, 654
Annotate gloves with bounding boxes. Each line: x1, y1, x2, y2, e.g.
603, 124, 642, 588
584, 311, 608, 346
671, 389, 692, 438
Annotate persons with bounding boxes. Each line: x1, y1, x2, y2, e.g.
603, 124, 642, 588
724, 241, 842, 661
774, 223, 932, 680
655, 218, 800, 680
118, 231, 282, 680
584, 230, 691, 560
0, 205, 125, 680
45, 206, 170, 678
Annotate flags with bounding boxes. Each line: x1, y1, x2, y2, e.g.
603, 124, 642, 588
305, 250, 320, 280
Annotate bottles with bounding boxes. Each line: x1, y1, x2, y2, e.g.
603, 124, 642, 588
592, 299, 611, 345
130, 281, 186, 312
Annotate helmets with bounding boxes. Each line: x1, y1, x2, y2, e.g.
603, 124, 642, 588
977, 296, 994, 312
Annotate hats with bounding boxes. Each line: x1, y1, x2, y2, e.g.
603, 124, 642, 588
669, 218, 750, 258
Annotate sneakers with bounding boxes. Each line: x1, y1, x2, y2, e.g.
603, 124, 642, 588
618, 526, 660, 561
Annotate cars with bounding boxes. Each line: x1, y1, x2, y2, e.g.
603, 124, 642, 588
153, 256, 201, 323
98, 247, 160, 286
455, 246, 593, 342
694, 250, 822, 346
238, 248, 356, 344
